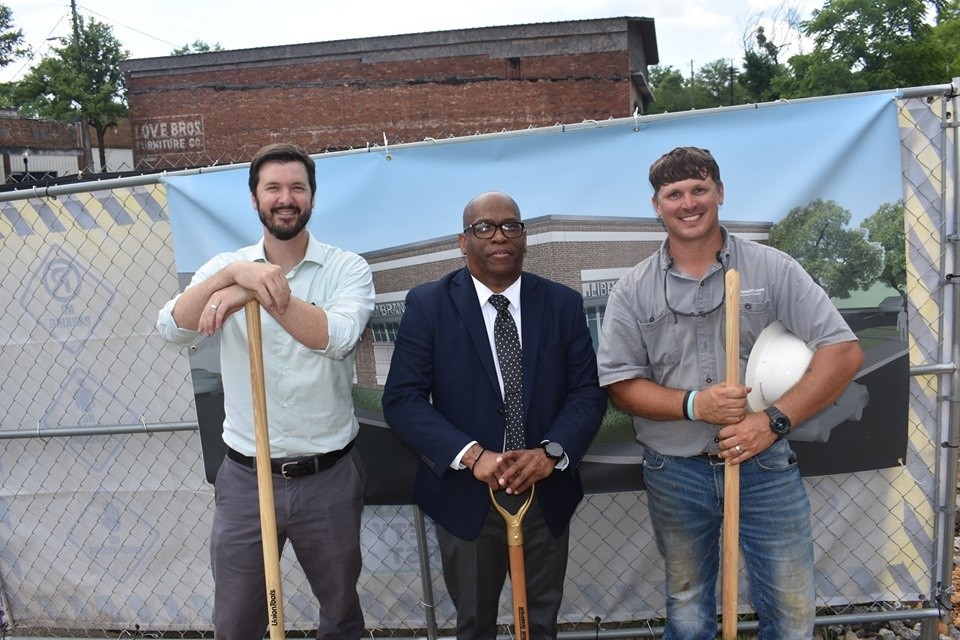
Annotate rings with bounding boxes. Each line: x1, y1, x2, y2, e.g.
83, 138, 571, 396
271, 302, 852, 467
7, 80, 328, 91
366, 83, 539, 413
210, 304, 218, 309
735, 445, 743, 454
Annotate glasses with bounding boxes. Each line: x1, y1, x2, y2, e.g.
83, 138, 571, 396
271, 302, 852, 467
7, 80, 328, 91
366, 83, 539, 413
463, 222, 525, 239
664, 249, 726, 317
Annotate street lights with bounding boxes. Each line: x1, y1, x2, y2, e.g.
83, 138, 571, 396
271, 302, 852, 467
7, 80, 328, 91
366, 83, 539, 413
46, 36, 94, 173
22, 152, 29, 181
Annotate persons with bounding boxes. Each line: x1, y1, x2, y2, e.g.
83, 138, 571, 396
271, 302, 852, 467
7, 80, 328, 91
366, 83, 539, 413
381, 192, 608, 640
595, 145, 867, 640
153, 143, 377, 640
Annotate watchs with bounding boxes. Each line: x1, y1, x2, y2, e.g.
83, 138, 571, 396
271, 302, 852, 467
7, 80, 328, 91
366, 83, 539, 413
539, 441, 564, 462
763, 405, 791, 444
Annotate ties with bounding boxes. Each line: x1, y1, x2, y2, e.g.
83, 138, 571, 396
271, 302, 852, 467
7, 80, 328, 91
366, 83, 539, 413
488, 295, 526, 452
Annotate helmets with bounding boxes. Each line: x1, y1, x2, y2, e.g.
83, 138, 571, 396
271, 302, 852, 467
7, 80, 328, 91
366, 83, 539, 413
743, 320, 814, 415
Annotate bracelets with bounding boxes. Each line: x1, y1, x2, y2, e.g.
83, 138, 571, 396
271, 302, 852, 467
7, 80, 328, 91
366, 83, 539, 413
687, 391, 699, 422
470, 447, 486, 473
682, 390, 691, 421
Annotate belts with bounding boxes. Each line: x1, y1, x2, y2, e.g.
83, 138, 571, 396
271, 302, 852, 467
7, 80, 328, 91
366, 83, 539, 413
227, 437, 355, 480
